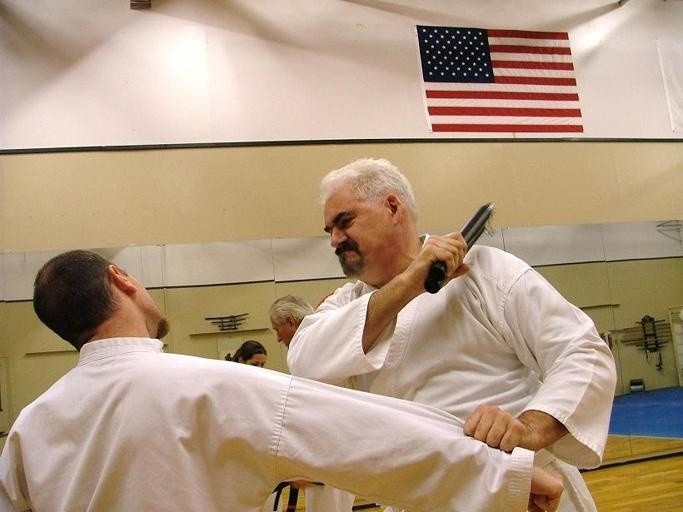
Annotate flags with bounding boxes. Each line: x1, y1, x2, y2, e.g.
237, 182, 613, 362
417, 25, 584, 133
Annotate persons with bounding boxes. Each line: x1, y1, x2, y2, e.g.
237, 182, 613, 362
225, 341, 284, 512
270, 295, 356, 512
0, 250, 563, 512
287, 158, 618, 512
